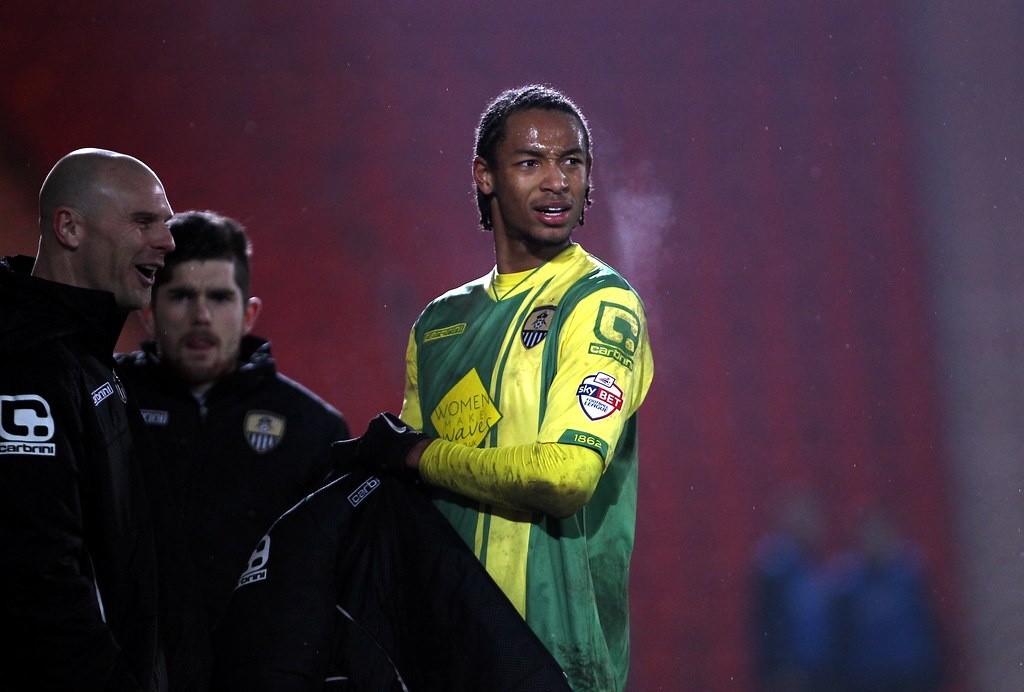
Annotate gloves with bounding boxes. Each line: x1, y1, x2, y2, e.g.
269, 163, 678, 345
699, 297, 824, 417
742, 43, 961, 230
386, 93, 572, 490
330, 411, 429, 474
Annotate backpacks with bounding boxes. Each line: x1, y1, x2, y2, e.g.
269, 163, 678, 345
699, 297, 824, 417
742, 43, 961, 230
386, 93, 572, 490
211, 436, 575, 692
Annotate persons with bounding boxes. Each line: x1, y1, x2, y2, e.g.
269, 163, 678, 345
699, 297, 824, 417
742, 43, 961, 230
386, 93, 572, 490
109, 211, 349, 692
330, 88, 655, 692
0, 146, 175, 692
